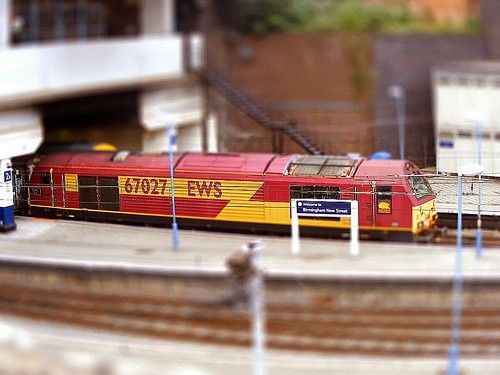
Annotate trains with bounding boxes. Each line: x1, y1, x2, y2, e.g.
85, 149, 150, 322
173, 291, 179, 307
13, 152, 437, 242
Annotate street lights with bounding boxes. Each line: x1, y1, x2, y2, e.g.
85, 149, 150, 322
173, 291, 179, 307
448, 164, 484, 374
